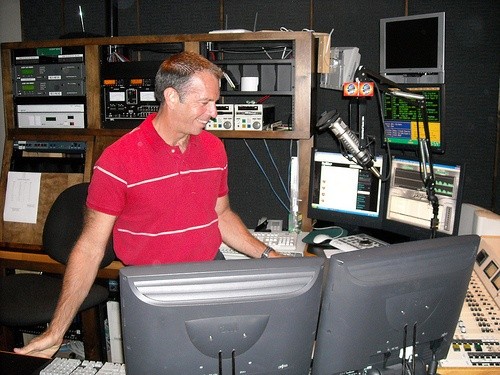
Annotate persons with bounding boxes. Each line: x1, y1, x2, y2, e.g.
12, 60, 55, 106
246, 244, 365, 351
14, 51, 293, 360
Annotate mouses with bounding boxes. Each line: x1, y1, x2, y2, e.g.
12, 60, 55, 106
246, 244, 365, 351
314, 235, 334, 245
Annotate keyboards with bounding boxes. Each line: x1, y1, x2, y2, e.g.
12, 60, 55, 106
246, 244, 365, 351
329, 233, 390, 252
218, 232, 297, 255
39, 357, 126, 375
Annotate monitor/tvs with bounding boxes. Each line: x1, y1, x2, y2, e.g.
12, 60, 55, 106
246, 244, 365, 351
384, 153, 465, 240
120, 256, 325, 375
307, 147, 388, 230
311, 234, 481, 375
381, 12, 445, 154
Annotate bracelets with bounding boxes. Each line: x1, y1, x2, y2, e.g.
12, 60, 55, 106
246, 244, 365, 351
257, 247, 275, 261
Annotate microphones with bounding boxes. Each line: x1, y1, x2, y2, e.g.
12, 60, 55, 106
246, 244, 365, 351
329, 117, 381, 177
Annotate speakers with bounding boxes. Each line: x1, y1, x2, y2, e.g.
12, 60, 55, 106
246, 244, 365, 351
472, 211, 500, 236
459, 202, 482, 235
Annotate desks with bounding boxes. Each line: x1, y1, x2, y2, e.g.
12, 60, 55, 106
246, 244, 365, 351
0, 229, 500, 375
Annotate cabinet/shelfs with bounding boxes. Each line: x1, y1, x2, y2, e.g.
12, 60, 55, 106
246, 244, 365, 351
0, 29, 319, 252
